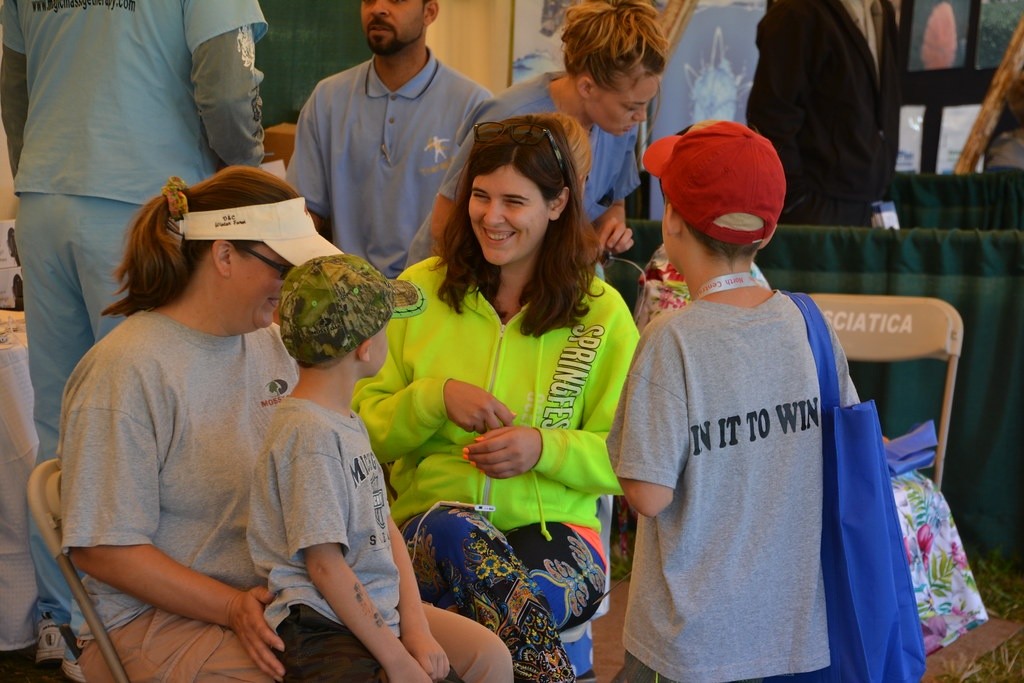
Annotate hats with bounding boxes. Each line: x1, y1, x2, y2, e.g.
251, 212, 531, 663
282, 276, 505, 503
643, 119, 788, 243
177, 196, 350, 267
273, 254, 427, 366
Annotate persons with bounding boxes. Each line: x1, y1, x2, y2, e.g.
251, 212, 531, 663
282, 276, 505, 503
348, 115, 641, 683
246, 254, 462, 683
400, 0, 672, 276
526, 112, 592, 204
623, 0, 906, 229
55, 165, 347, 683
606, 121, 863, 682
986, 70, 1024, 168
287, 0, 496, 281
1, 1, 270, 682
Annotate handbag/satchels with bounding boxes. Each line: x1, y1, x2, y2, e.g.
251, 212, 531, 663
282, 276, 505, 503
783, 288, 927, 683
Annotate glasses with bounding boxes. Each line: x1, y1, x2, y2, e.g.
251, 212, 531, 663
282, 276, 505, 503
231, 241, 293, 280
471, 120, 566, 178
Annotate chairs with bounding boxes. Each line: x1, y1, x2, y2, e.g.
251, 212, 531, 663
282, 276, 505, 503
809, 293, 963, 490
26, 458, 129, 683
559, 493, 613, 643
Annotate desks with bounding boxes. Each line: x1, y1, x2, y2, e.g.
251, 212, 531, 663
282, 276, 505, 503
0, 309, 44, 651
